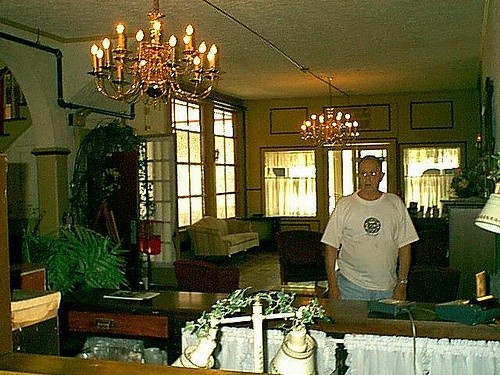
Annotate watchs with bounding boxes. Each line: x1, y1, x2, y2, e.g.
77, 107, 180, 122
398, 279, 408, 285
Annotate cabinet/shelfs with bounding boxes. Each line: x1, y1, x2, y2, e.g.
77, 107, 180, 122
61, 287, 500, 375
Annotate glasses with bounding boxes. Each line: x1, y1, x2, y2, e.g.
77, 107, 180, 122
359, 171, 383, 177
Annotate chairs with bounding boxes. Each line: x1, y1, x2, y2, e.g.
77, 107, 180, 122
174, 259, 240, 294
276, 230, 328, 288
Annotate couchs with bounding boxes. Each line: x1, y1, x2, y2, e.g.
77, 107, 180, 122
187, 217, 260, 257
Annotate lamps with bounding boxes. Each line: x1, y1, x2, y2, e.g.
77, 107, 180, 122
171, 287, 335, 375
86, 1, 219, 112
475, 182, 500, 233
299, 76, 360, 144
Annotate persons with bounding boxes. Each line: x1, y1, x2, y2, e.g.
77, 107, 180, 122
320, 155, 420, 302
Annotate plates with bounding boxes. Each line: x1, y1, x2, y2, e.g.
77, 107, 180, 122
103, 290, 160, 300
